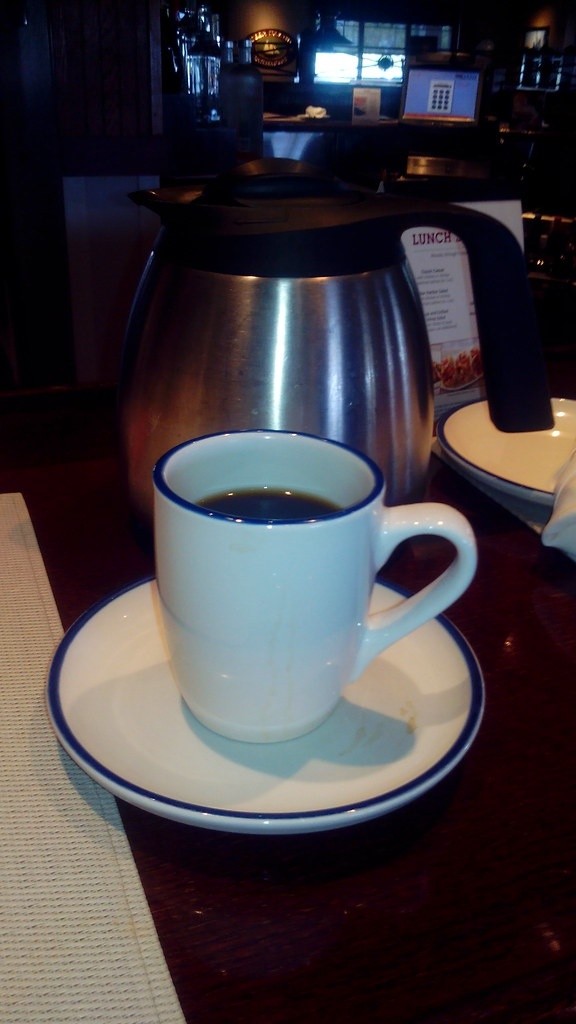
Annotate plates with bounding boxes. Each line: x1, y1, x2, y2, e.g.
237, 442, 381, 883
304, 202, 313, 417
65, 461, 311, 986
437, 396, 576, 508
442, 372, 482, 392
46, 578, 484, 834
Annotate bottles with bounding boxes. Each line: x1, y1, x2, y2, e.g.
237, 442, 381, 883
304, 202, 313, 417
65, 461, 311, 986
523, 208, 576, 296
167, 0, 265, 165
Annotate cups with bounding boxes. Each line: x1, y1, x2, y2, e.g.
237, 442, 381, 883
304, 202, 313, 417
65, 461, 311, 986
152, 431, 478, 744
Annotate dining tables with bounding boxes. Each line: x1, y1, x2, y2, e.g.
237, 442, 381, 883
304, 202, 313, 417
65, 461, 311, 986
0, 373, 576, 1024
263, 113, 399, 159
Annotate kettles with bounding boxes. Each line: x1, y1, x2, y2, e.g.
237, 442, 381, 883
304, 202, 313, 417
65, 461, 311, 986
121, 181, 557, 530
